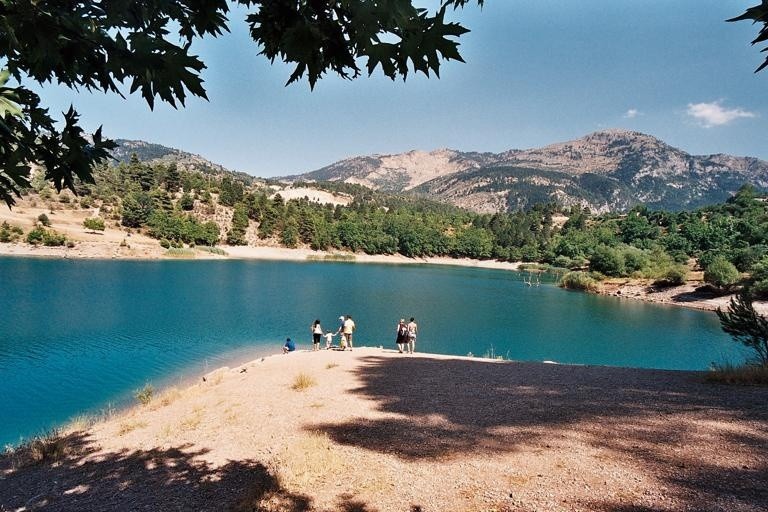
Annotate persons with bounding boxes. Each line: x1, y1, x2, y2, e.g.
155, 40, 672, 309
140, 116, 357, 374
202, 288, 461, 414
322, 330, 338, 349
343, 315, 355, 351
282, 338, 295, 352
339, 330, 347, 351
311, 319, 323, 351
396, 319, 408, 353
336, 315, 344, 332
406, 317, 417, 354
